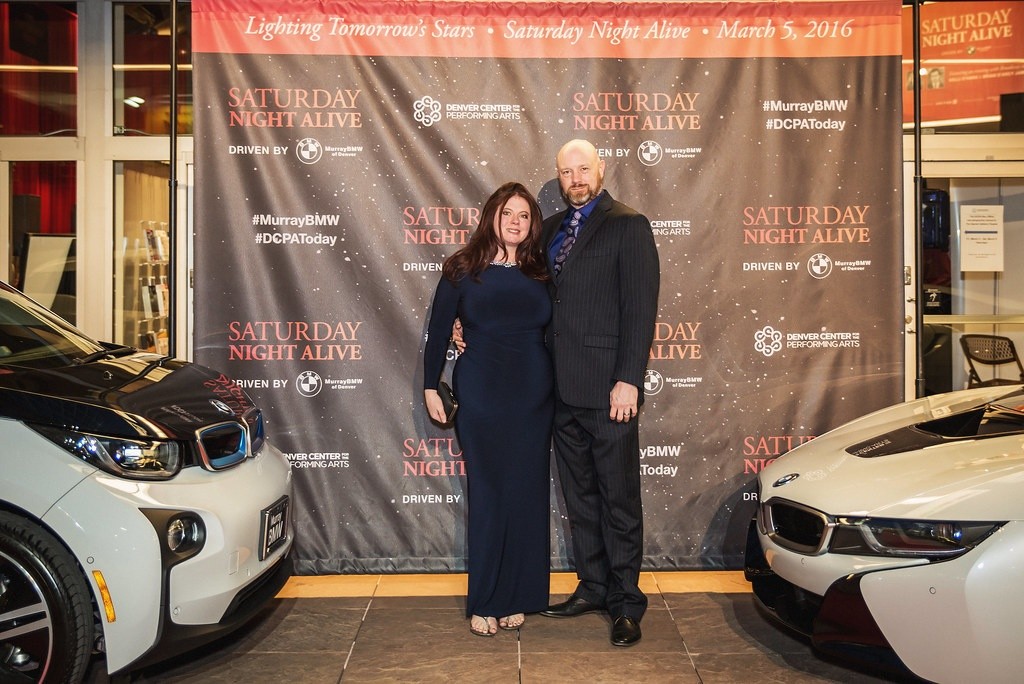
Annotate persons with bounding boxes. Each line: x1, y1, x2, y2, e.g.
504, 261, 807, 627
451, 139, 661, 647
423, 181, 557, 635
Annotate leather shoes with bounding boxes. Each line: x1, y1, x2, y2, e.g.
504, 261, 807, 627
611, 615, 642, 647
540, 595, 607, 619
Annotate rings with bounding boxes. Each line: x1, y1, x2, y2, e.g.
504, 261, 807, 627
624, 413, 629, 415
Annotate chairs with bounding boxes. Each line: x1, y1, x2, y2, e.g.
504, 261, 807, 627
959, 334, 1024, 390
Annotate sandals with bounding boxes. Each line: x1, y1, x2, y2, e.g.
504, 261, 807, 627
470, 614, 525, 637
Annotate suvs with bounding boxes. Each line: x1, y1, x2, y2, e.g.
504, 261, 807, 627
0, 278, 295, 684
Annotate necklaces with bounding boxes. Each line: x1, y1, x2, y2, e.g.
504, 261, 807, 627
487, 260, 517, 267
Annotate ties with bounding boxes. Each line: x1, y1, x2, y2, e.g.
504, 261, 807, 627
554, 210, 581, 276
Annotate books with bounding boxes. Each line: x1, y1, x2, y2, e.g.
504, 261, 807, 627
138, 229, 171, 356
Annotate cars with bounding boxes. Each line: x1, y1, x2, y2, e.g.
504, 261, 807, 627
744, 384, 1024, 684
